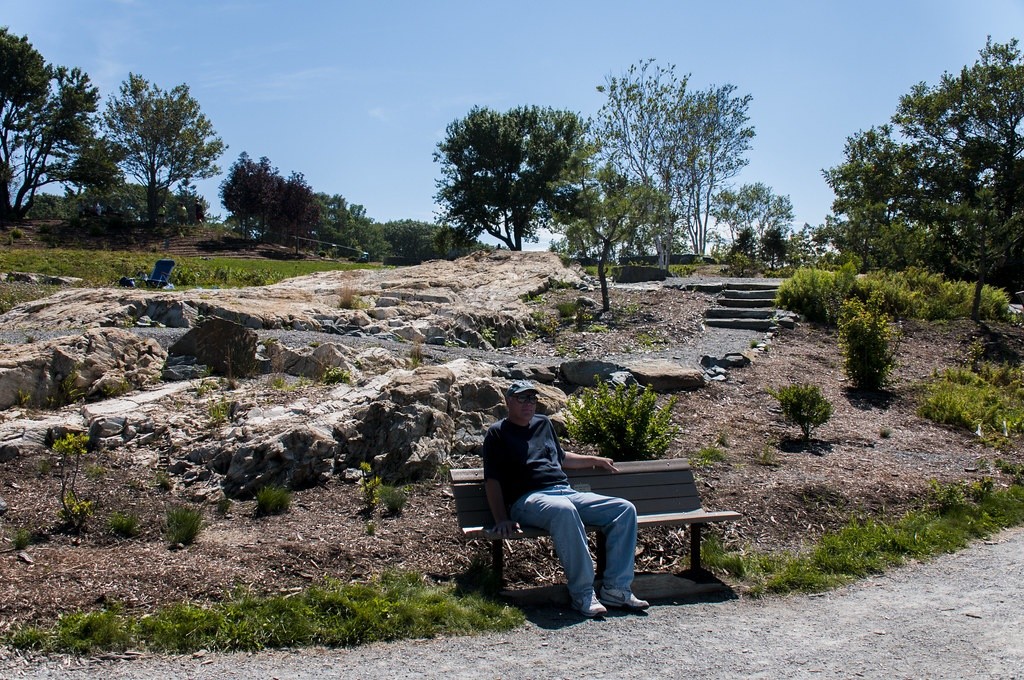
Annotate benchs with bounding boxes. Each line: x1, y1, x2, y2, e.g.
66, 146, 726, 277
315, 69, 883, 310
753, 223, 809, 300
449, 458, 742, 617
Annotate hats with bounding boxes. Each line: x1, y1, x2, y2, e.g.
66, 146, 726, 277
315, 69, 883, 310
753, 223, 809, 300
505, 381, 539, 400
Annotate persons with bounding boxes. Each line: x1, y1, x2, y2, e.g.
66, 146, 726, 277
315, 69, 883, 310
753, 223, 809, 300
482, 380, 650, 617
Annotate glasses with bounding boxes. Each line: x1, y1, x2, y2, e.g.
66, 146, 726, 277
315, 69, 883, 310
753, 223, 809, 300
509, 393, 538, 405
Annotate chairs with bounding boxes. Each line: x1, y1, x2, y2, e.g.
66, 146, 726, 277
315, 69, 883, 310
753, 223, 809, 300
120, 259, 175, 288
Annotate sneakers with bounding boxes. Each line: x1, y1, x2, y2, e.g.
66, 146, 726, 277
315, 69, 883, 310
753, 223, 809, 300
571, 589, 607, 617
598, 586, 649, 610
